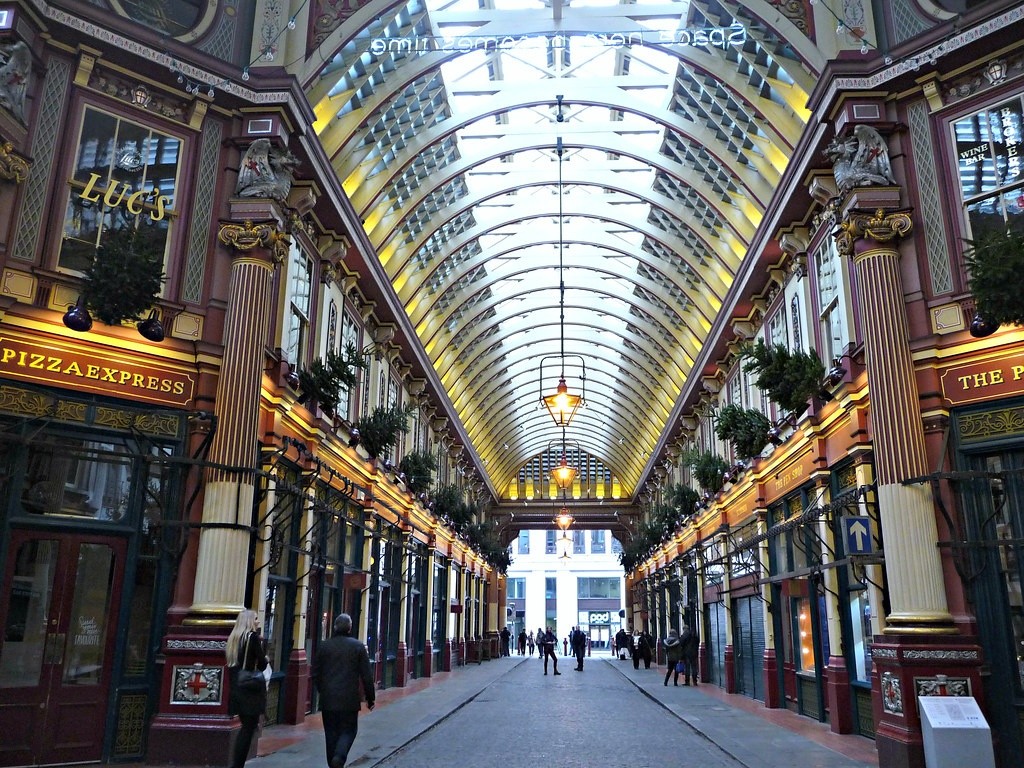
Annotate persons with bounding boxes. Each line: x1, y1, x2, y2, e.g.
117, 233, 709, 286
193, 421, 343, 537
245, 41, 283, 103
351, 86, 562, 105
311, 614, 376, 768
225, 610, 268, 768
500, 625, 700, 686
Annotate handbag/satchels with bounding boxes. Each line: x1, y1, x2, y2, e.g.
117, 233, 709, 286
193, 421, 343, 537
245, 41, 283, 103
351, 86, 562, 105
238, 670, 265, 690
676, 662, 686, 673
650, 648, 655, 656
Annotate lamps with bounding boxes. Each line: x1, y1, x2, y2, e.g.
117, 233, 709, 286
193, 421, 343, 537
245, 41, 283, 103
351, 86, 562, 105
550, 426, 578, 490
540, 280, 581, 490
132, 307, 165, 341
970, 304, 1000, 338
553, 490, 576, 530
539, 138, 584, 426
60, 281, 93, 332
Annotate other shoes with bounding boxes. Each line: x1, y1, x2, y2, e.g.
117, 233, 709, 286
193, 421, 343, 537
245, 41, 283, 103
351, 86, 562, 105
682, 683, 690, 686
331, 753, 344, 768
664, 683, 667, 686
674, 683, 677, 686
694, 683, 697, 686
